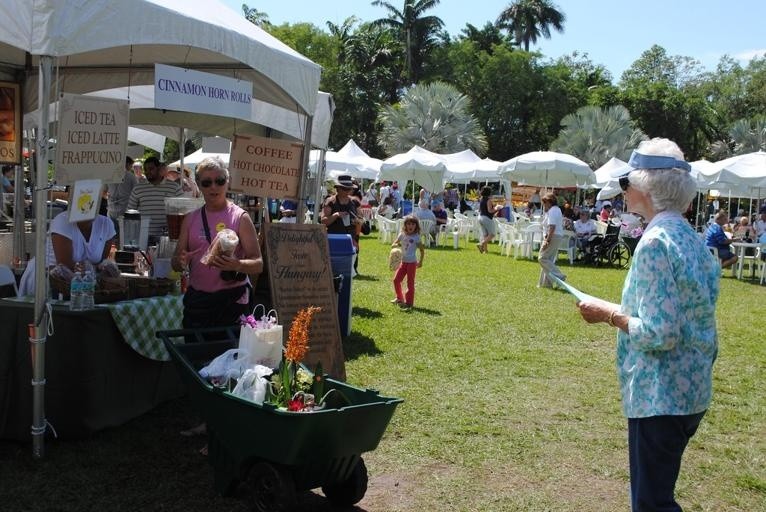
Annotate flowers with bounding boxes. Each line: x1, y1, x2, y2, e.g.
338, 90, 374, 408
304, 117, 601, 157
265, 295, 330, 412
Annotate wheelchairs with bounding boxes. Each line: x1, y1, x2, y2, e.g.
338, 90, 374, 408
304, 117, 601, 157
586, 221, 632, 269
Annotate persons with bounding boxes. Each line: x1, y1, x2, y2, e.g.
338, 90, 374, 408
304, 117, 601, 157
170, 153, 266, 458
303, 139, 507, 272
573, 138, 722, 512
537, 193, 567, 288
388, 215, 426, 313
2, 141, 309, 225
14, 207, 130, 301
498, 148, 766, 270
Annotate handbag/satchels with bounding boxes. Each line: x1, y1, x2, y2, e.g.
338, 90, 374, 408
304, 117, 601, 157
237, 304, 283, 371
361, 217, 370, 235
221, 270, 246, 281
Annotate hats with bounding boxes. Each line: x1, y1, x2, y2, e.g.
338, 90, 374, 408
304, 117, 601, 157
334, 176, 358, 189
610, 148, 691, 177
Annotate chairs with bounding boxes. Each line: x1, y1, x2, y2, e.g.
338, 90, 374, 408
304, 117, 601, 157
365, 195, 764, 285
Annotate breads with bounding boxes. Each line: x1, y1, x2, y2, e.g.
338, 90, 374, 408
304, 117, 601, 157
208, 229, 238, 268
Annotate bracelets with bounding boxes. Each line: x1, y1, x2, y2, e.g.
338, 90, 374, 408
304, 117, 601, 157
606, 308, 621, 328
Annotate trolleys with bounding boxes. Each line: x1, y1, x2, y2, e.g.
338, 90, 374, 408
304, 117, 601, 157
154, 321, 408, 511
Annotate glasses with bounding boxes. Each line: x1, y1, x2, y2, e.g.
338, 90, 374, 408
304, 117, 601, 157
619, 178, 630, 191
201, 176, 225, 188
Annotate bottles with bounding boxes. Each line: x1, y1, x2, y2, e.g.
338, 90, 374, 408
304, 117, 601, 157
68, 271, 82, 312
159, 232, 171, 258
180, 269, 187, 295
107, 245, 116, 262
82, 271, 94, 310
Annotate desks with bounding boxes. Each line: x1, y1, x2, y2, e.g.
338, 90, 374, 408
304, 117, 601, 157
1, 283, 197, 445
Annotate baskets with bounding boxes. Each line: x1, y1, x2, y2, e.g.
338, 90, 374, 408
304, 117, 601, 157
50, 270, 172, 304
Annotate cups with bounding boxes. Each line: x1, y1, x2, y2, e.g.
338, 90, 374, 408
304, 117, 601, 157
249, 200, 255, 206
166, 271, 181, 295
341, 213, 350, 227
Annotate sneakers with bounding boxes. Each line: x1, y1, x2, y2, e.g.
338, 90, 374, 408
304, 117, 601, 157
554, 275, 567, 288
477, 242, 484, 253
390, 298, 404, 303
